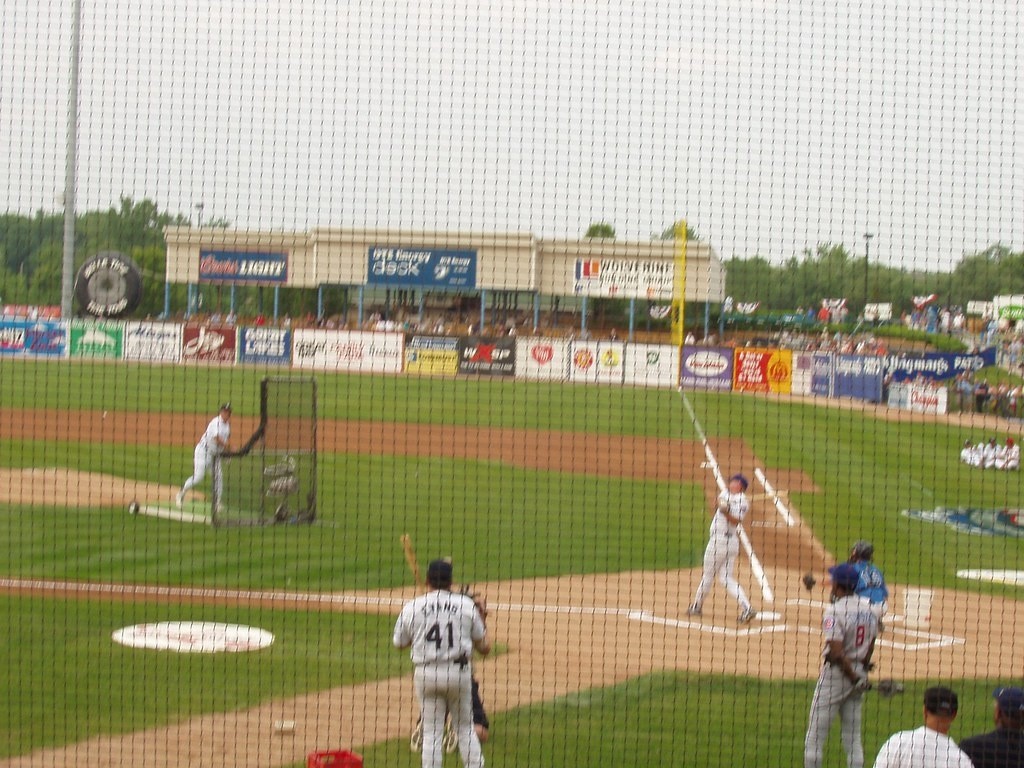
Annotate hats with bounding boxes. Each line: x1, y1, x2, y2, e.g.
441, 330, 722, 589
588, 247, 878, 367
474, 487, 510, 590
924, 684, 959, 712
828, 564, 859, 585
1006, 438, 1014, 444
221, 402, 231, 409
993, 688, 1024, 713
427, 560, 452, 581
988, 437, 996, 443
731, 474, 748, 490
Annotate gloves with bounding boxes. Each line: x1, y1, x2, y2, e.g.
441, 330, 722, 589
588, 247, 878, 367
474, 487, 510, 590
717, 497, 729, 513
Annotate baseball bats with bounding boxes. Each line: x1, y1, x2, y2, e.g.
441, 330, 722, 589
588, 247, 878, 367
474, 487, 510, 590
727, 488, 789, 508
863, 682, 904, 694
399, 531, 424, 595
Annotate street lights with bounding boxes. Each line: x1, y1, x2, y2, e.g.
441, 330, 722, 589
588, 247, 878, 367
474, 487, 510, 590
864, 234, 873, 307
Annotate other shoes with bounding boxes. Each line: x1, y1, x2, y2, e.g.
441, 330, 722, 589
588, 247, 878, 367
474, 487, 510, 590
687, 606, 702, 616
473, 723, 489, 742
176, 493, 183, 509
736, 608, 756, 623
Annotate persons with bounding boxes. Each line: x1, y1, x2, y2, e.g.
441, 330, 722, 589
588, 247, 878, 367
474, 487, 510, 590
688, 474, 757, 624
307, 304, 620, 342
393, 559, 493, 768
685, 327, 888, 355
873, 686, 975, 768
794, 302, 848, 323
961, 437, 1021, 471
256, 308, 292, 327
901, 304, 1024, 415
804, 563, 878, 768
201, 307, 238, 324
958, 686, 1024, 768
176, 402, 232, 513
848, 540, 888, 662
